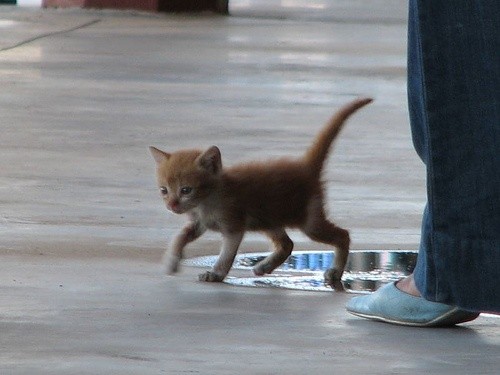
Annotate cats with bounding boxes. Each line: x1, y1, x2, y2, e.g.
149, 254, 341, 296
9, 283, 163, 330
147, 98, 374, 282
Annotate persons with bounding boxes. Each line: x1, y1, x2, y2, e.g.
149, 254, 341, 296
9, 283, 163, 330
343, 1, 500, 327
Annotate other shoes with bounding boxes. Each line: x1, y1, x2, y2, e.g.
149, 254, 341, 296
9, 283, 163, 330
344, 276, 479, 328
